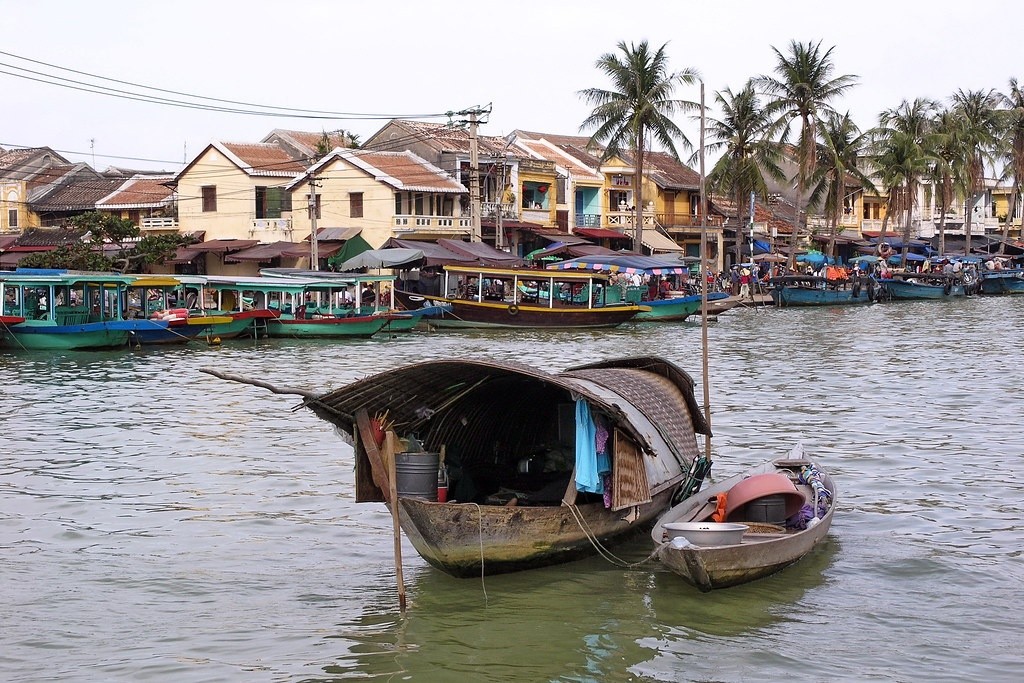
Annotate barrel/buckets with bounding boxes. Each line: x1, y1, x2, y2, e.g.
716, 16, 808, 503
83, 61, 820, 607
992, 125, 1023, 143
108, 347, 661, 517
438, 487, 448, 502
394, 453, 440, 503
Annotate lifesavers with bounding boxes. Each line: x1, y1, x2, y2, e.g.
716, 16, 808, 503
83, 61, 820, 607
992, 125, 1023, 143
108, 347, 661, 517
508, 304, 518, 314
869, 285, 874, 301
150, 310, 167, 321
969, 284, 980, 294
665, 294, 684, 298
944, 284, 952, 295
665, 291, 684, 294
163, 309, 190, 316
878, 243, 892, 254
775, 284, 784, 291
965, 285, 969, 295
162, 313, 188, 320
853, 285, 860, 297
168, 318, 185, 322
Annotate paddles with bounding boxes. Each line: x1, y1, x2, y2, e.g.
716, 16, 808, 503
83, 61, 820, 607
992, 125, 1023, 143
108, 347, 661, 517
386, 430, 406, 616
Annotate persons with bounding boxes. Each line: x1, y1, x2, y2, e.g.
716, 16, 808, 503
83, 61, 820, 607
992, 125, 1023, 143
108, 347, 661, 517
706, 256, 968, 299
361, 284, 375, 304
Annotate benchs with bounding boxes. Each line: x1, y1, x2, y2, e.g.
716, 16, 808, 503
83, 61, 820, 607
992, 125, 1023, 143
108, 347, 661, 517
484, 291, 537, 303
4, 302, 89, 326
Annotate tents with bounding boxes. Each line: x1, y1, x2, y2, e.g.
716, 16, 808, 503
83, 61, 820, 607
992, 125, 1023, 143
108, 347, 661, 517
727, 244, 981, 276
378, 237, 646, 292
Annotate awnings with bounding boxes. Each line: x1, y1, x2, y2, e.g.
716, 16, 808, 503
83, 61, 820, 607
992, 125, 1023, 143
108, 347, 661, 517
573, 228, 627, 239
520, 227, 593, 247
621, 229, 684, 253
745, 231, 900, 245
150, 227, 363, 265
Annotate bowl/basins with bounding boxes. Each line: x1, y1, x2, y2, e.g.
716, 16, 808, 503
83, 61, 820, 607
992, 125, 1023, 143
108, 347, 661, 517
661, 522, 749, 545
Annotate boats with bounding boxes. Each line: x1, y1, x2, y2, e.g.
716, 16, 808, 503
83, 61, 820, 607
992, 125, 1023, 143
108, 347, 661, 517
652, 442, 837, 591
257, 267, 453, 332
188, 280, 282, 338
0, 315, 25, 329
306, 354, 713, 576
75, 276, 233, 342
0, 269, 168, 350
516, 254, 729, 322
201, 275, 413, 338
692, 296, 744, 314
394, 264, 652, 328
875, 279, 979, 299
981, 268, 1024, 293
765, 275, 881, 306
